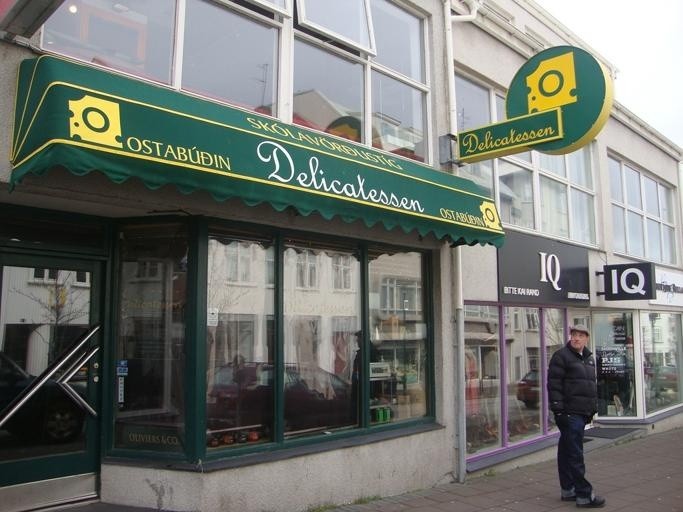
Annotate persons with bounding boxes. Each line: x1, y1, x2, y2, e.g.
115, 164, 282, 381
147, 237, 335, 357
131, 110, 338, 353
544, 324, 607, 509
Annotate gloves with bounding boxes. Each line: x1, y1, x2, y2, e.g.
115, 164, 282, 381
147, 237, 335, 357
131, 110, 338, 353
555, 413, 568, 429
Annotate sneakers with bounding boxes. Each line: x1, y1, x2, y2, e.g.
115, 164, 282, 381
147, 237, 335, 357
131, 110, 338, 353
578, 495, 605, 507
562, 495, 576, 501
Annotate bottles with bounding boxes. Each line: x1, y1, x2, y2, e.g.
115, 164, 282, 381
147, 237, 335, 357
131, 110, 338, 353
370, 397, 399, 425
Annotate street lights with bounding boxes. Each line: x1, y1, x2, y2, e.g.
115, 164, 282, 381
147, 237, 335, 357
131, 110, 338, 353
399, 289, 409, 396
647, 311, 662, 410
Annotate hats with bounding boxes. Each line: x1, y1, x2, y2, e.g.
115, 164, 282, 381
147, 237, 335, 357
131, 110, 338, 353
570, 324, 589, 335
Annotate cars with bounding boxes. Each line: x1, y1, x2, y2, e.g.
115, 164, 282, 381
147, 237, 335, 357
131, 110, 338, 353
0, 347, 86, 446
203, 354, 352, 431
515, 345, 677, 407
113, 313, 184, 400
